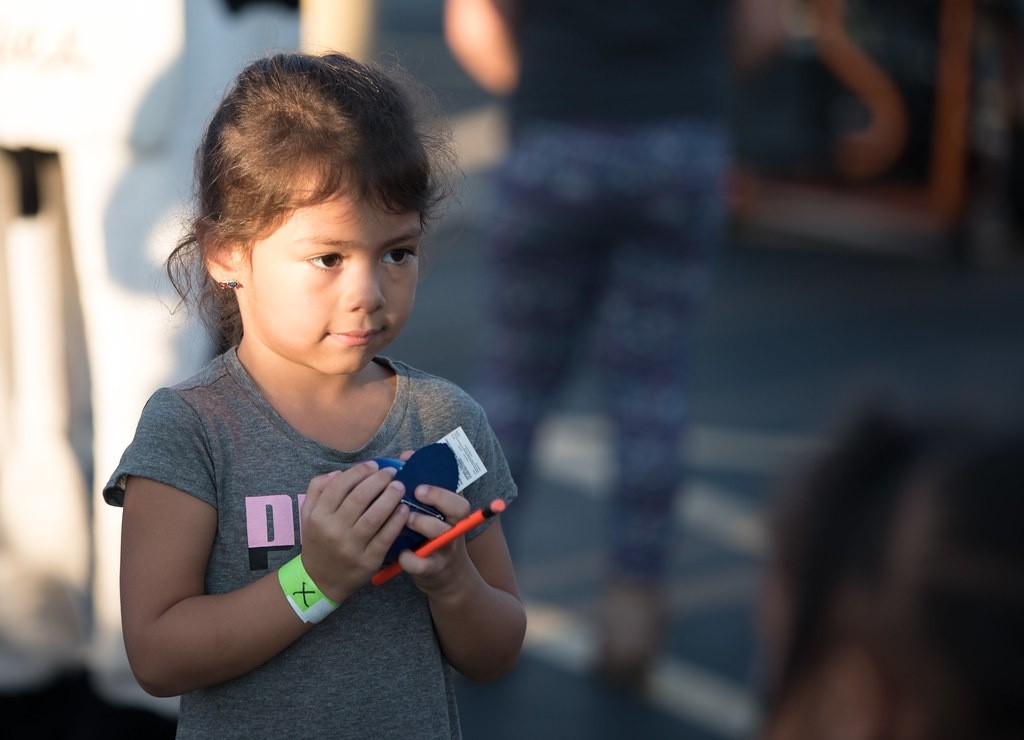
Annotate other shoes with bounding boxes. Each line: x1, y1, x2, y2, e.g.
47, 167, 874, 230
575, 639, 668, 710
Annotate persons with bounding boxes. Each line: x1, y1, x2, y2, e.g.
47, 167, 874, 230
0, 1, 231, 740
440, 0, 783, 699
99, 53, 527, 740
747, 393, 1024, 740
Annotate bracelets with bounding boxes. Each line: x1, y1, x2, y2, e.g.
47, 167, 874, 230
277, 554, 341, 625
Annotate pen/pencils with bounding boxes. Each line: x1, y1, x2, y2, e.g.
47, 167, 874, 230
371, 498, 507, 586
